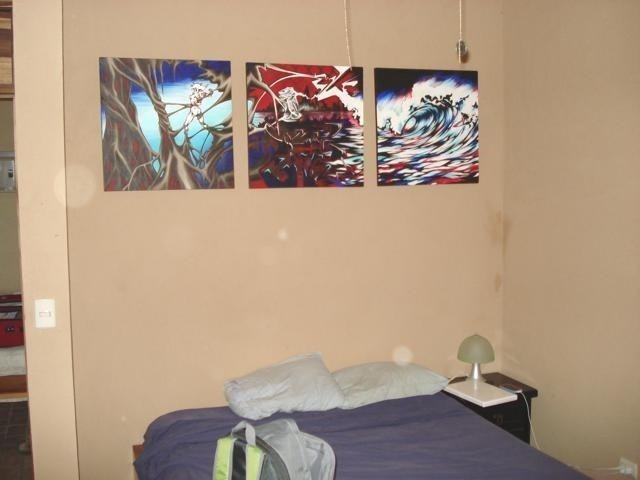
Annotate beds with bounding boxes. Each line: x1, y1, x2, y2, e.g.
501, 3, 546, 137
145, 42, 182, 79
131, 393, 599, 480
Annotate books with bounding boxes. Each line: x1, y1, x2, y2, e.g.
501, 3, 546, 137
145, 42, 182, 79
437, 379, 518, 408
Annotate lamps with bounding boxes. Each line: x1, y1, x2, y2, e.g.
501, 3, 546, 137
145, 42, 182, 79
457, 333, 495, 383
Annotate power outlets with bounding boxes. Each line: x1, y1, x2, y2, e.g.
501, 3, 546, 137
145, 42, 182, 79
619, 457, 637, 479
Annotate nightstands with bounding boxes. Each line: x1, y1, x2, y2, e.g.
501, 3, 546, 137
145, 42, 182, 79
441, 372, 538, 446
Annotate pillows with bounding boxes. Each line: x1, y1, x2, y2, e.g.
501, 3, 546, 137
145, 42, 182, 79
329, 361, 450, 409
223, 352, 345, 420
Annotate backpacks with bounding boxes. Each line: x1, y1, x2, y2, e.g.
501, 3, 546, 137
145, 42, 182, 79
213, 417, 336, 480
